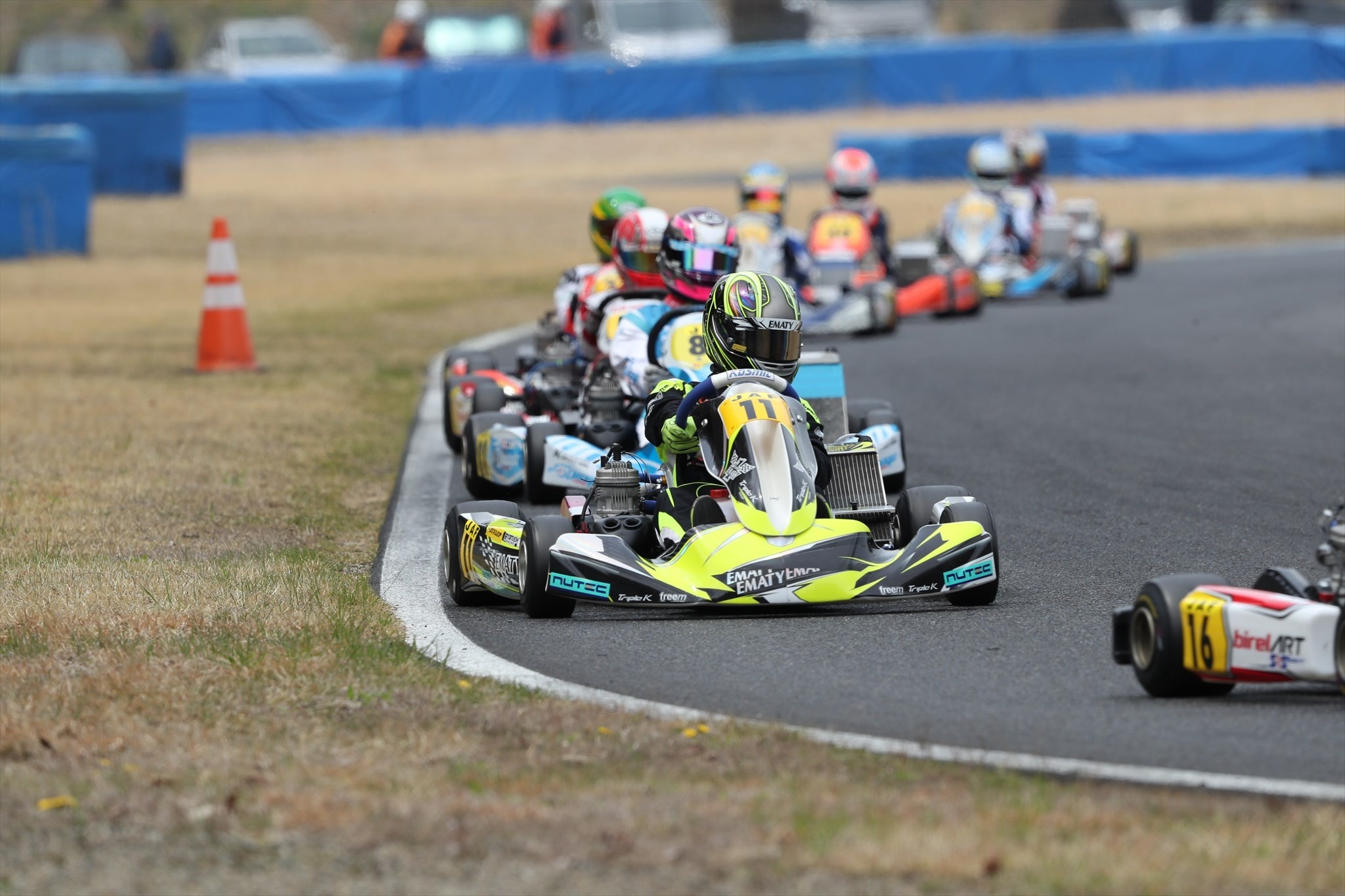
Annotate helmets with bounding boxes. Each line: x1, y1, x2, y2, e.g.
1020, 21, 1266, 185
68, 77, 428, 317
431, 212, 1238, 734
1006, 122, 1048, 173
590, 187, 647, 261
704, 270, 804, 384
969, 140, 1015, 189
741, 161, 791, 211
829, 145, 879, 194
810, 212, 870, 260
656, 206, 742, 300
609, 206, 672, 295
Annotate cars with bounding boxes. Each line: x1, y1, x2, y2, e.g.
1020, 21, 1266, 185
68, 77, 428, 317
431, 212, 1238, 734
424, 7, 529, 60
8, 28, 136, 81
550, 0, 931, 63
1044, 0, 1343, 33
202, 16, 349, 72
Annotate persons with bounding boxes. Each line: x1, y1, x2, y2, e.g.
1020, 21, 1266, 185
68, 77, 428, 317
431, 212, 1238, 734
553, 189, 741, 450
375, 0, 430, 63
809, 148, 899, 277
644, 271, 834, 552
142, 7, 182, 72
531, 0, 576, 58
740, 162, 820, 308
939, 130, 1058, 275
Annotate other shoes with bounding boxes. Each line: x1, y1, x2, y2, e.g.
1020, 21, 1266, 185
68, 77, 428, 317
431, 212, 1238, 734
815, 493, 834, 518
691, 493, 729, 527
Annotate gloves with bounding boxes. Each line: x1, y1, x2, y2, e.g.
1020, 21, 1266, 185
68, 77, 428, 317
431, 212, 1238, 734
663, 412, 699, 452
644, 364, 674, 392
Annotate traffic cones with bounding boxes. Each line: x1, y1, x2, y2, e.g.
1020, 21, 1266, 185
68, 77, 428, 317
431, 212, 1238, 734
177, 216, 270, 376
